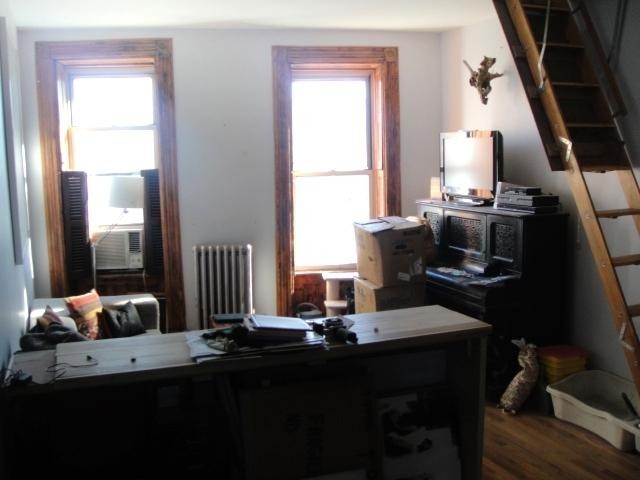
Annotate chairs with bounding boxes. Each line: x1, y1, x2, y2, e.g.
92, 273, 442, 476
30, 293, 162, 340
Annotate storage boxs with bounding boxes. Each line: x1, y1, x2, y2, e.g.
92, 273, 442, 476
237, 362, 373, 479
353, 277, 426, 314
354, 216, 438, 288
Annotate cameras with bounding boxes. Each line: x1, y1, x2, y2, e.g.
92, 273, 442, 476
313, 316, 359, 346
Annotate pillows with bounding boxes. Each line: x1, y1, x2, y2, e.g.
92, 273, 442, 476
64, 288, 112, 340
37, 305, 63, 328
102, 302, 146, 338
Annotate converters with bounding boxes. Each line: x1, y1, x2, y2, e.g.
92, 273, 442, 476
10, 372, 32, 386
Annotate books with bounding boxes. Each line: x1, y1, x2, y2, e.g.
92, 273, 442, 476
244, 314, 314, 337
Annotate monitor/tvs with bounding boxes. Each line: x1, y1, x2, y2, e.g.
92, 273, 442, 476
440, 130, 503, 207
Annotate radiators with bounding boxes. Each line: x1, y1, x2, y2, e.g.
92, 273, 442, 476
191, 244, 255, 329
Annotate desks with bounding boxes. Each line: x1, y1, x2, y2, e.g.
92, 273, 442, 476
3, 304, 494, 480
415, 198, 570, 407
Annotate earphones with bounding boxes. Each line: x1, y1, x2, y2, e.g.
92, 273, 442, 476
131, 358, 136, 362
87, 355, 92, 361
